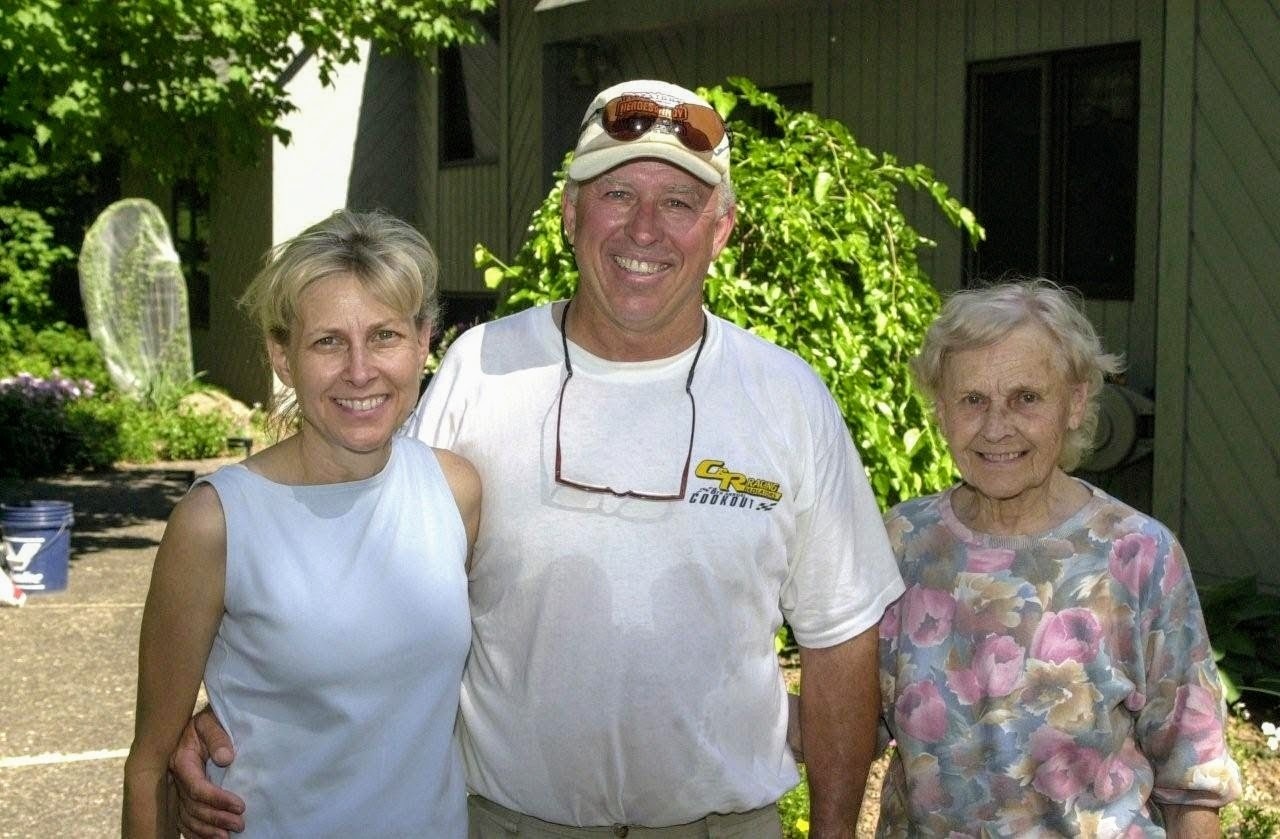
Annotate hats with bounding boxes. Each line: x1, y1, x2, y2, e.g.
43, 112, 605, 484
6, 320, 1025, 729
567, 79, 730, 187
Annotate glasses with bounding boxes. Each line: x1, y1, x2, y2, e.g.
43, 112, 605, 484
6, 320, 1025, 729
554, 376, 697, 501
577, 95, 734, 154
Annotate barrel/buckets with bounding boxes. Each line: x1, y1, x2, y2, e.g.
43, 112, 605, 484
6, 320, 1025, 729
0, 500, 74, 592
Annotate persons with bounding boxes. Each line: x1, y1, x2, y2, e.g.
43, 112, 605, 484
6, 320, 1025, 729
166, 80, 906, 838
120, 208, 488, 838
789, 275, 1243, 839
